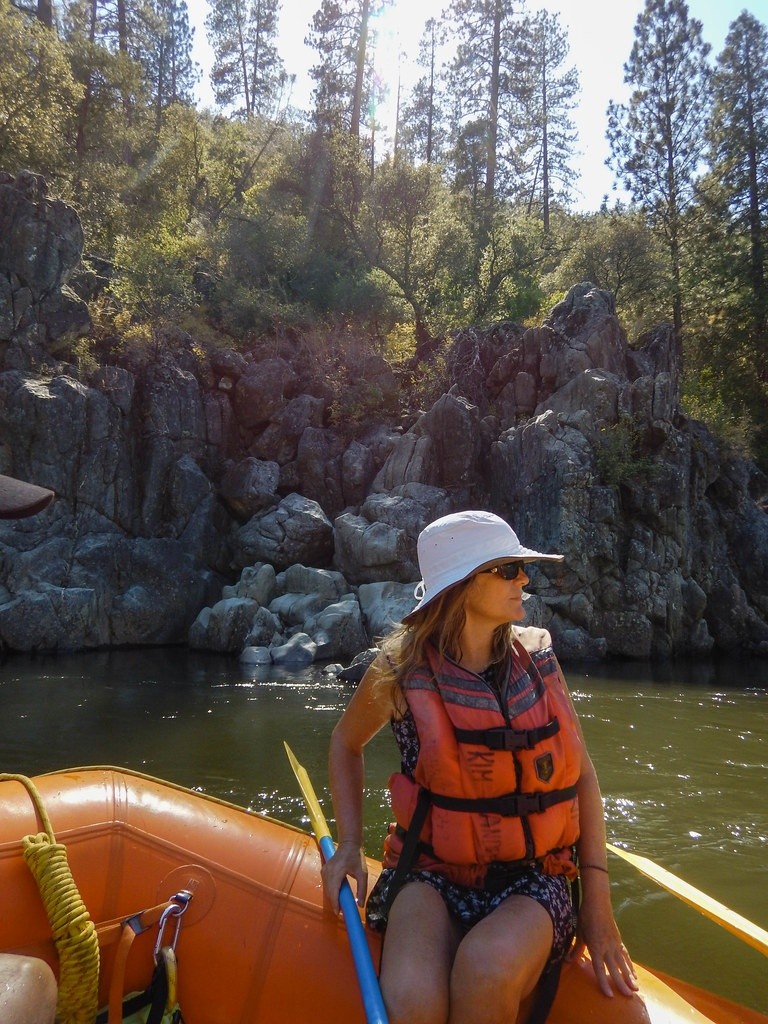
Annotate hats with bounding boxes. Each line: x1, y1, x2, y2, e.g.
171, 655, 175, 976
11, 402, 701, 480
400, 511, 565, 626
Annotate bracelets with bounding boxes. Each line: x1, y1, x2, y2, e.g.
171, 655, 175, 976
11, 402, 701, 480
579, 864, 609, 875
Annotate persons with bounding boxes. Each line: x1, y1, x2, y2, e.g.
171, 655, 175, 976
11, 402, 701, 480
321, 507, 644, 1024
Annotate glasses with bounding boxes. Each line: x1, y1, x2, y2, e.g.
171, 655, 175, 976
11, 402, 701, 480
479, 560, 525, 581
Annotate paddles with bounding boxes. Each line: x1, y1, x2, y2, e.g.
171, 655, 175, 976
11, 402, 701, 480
282, 739, 390, 1023
605, 841, 768, 961
